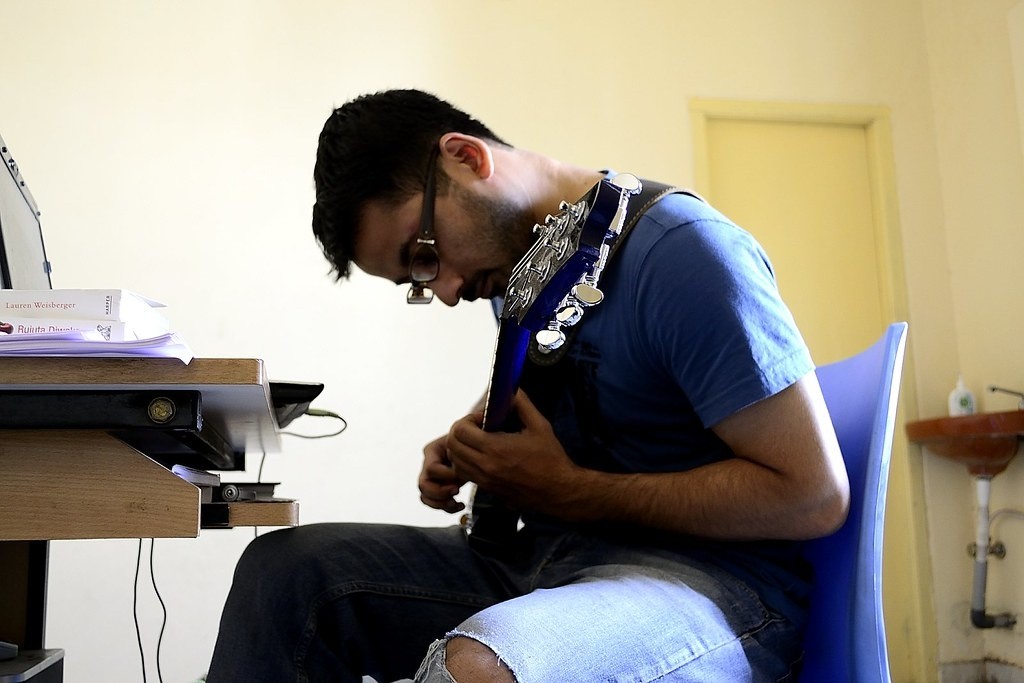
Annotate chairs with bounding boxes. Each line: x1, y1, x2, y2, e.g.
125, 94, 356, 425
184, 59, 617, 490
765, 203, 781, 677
799, 319, 907, 683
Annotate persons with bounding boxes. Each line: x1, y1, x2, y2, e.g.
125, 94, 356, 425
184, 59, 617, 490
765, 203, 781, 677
205, 89, 851, 683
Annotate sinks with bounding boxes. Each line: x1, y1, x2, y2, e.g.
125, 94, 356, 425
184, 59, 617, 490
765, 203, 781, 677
904, 413, 1024, 479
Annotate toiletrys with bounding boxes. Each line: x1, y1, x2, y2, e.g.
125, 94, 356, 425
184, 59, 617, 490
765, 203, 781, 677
948, 374, 977, 417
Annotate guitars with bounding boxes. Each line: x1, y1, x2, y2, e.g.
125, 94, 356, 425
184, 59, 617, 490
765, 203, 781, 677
457, 171, 644, 534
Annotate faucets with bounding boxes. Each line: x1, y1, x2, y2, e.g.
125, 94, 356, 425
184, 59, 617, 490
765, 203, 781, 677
988, 383, 1024, 410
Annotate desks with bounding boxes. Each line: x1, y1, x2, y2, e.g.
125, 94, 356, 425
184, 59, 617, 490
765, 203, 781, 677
0, 355, 300, 683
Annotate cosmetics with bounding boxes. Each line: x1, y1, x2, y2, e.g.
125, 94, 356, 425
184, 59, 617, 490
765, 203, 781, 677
947, 375, 976, 418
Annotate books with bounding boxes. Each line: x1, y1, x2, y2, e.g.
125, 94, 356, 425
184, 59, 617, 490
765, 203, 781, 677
171, 464, 221, 504
0, 288, 195, 366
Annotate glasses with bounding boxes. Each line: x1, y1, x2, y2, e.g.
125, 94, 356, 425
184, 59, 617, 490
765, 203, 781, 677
407, 177, 439, 302
